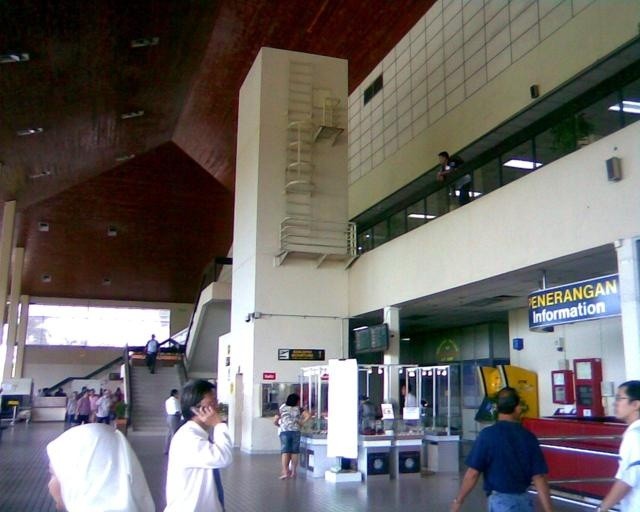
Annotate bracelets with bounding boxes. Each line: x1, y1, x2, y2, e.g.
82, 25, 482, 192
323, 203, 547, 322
453, 496, 464, 505
597, 504, 601, 512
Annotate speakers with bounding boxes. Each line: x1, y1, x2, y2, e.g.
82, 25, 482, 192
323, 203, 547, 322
607, 158, 621, 180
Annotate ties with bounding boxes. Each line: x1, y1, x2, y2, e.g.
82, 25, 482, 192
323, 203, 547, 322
209, 437, 225, 511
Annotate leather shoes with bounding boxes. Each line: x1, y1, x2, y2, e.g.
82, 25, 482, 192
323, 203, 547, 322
279, 475, 288, 481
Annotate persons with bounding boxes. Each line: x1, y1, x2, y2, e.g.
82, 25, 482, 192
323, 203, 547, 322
40, 388, 49, 396
144, 333, 161, 374
164, 388, 183, 454
273, 393, 312, 479
449, 386, 555, 512
595, 380, 640, 512
45, 421, 157, 511
55, 387, 64, 396
436, 151, 473, 204
160, 379, 235, 512
401, 383, 416, 407
66, 386, 124, 427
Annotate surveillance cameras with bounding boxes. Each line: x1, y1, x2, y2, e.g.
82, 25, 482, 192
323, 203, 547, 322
245, 316, 250, 322
